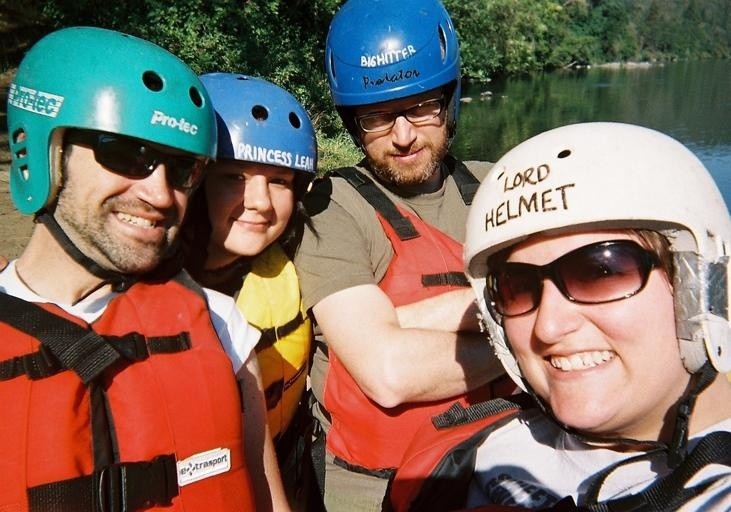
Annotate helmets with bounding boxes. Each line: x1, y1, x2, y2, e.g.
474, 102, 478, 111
199, 73, 318, 201
325, 1, 462, 148
462, 119, 731, 398
7, 25, 217, 216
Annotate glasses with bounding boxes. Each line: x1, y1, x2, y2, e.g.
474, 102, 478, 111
67, 131, 211, 190
354, 93, 448, 134
485, 238, 662, 318
